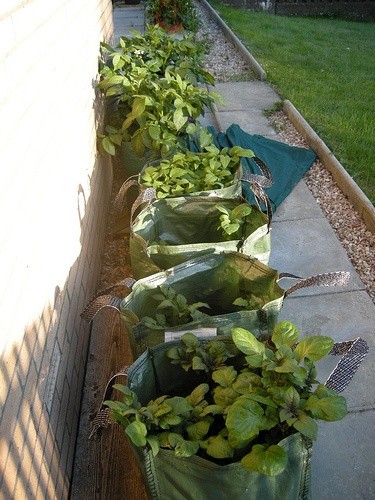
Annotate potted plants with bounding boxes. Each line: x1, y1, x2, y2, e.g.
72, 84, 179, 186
100, 27, 348, 500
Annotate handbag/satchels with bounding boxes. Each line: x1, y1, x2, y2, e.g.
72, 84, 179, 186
128, 181, 273, 279
111, 155, 272, 214
88, 336, 368, 499
81, 249, 350, 363
